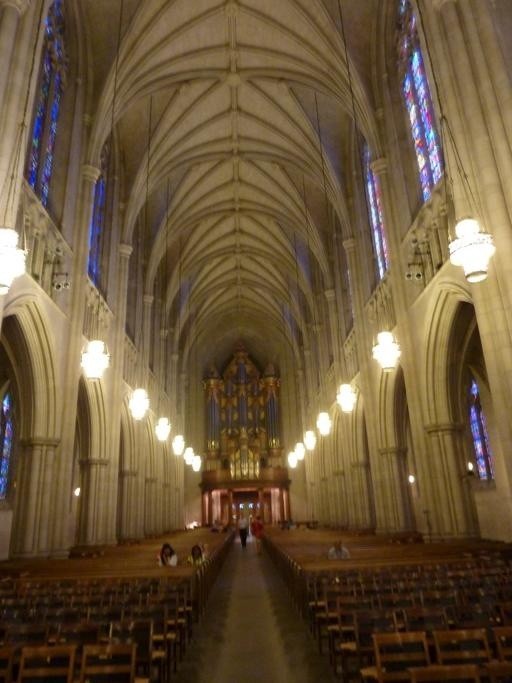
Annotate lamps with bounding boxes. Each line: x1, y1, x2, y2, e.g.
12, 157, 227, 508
2, 1, 46, 295
337, 1, 403, 373
78, 3, 125, 379
415, 1, 497, 285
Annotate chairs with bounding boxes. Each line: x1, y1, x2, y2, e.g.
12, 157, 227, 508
263, 522, 512, 683
0, 524, 239, 683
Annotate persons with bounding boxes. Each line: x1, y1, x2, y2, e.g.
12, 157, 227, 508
186, 545, 205, 566
238, 512, 247, 550
157, 542, 178, 566
328, 539, 351, 560
251, 516, 264, 553
